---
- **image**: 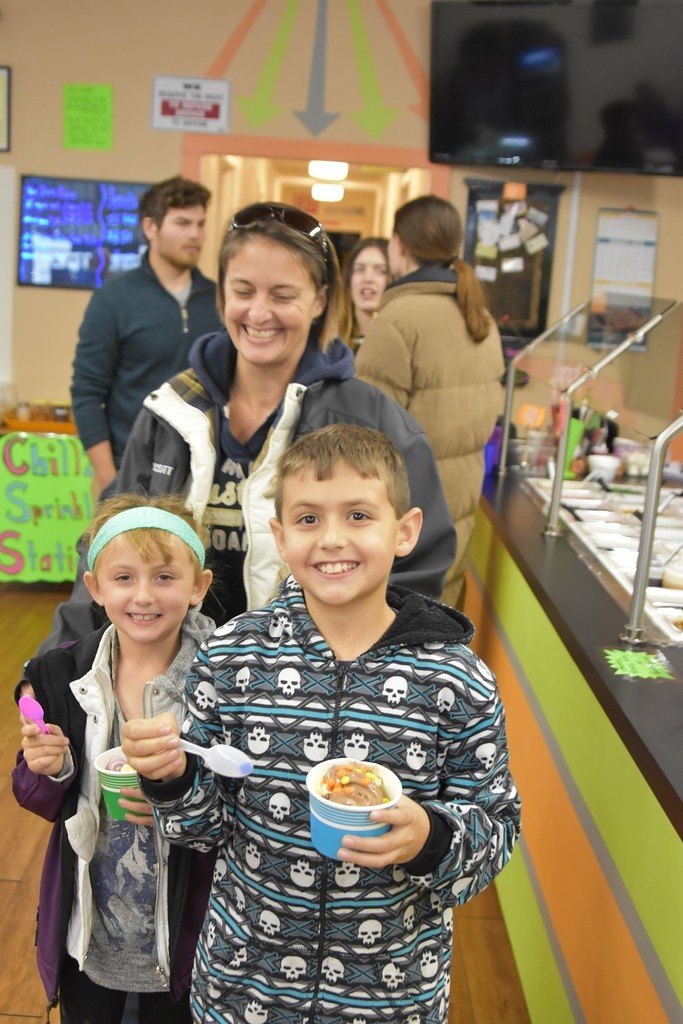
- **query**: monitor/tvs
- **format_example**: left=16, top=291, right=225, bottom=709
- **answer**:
left=428, top=0, right=683, bottom=177
left=17, top=175, right=155, bottom=291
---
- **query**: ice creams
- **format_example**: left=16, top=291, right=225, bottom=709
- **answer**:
left=321, top=761, right=390, bottom=807
left=105, top=756, right=137, bottom=774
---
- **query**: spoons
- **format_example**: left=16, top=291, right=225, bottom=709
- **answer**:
left=177, top=736, right=255, bottom=778
left=17, top=696, right=52, bottom=737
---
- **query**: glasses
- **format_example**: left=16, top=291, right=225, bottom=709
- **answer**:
left=226, top=206, right=330, bottom=270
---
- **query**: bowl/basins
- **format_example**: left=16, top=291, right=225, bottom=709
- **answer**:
left=305, top=758, right=402, bottom=862
left=93, top=746, right=150, bottom=822
left=588, top=454, right=620, bottom=481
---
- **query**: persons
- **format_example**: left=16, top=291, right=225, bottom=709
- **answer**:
left=121, top=423, right=524, bottom=1024
left=342, top=194, right=505, bottom=614
left=70, top=175, right=228, bottom=513
left=12, top=491, right=230, bottom=1024
left=38, top=201, right=458, bottom=659
